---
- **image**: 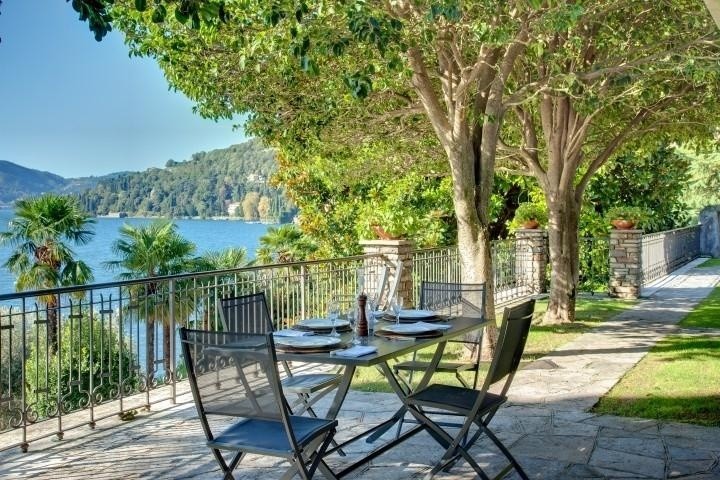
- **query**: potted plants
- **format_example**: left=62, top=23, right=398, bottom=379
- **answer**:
left=363, top=204, right=423, bottom=240
left=513, top=202, right=548, bottom=229
left=603, top=206, right=651, bottom=229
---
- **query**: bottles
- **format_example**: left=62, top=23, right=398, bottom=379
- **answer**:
left=355, top=275, right=373, bottom=338
left=357, top=295, right=368, bottom=345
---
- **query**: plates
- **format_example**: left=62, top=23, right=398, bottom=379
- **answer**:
left=275, top=345, right=347, bottom=355
left=298, top=319, right=350, bottom=330
left=292, top=327, right=352, bottom=333
left=384, top=324, right=431, bottom=335
left=389, top=310, right=436, bottom=319
left=374, top=330, right=443, bottom=338
left=383, top=316, right=442, bottom=322
left=276, top=336, right=342, bottom=349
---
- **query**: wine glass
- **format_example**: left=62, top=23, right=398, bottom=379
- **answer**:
left=328, top=308, right=341, bottom=337
left=393, top=297, right=404, bottom=326
